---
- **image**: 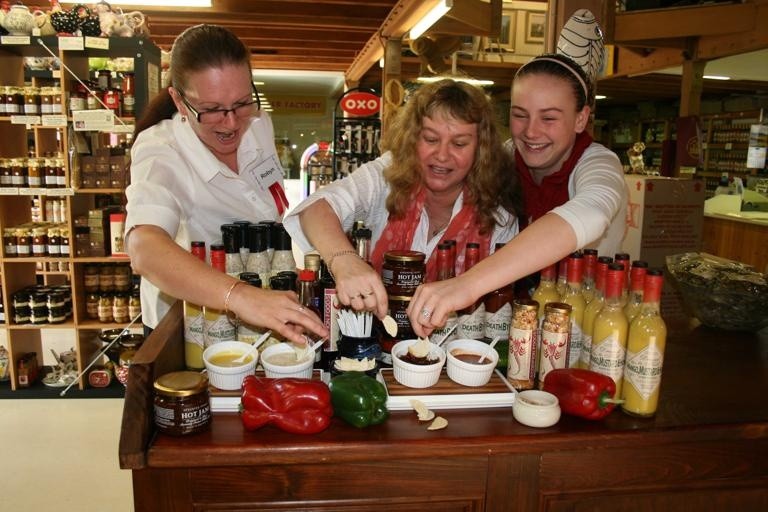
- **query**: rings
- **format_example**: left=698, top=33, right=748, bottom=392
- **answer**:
left=419, top=310, right=431, bottom=319
left=363, top=291, right=374, bottom=300
left=349, top=294, right=361, bottom=299
left=297, top=305, right=305, bottom=311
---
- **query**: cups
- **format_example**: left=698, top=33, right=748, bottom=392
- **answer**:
left=512, top=390, right=561, bottom=427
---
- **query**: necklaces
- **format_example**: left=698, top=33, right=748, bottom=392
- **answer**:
left=431, top=222, right=446, bottom=236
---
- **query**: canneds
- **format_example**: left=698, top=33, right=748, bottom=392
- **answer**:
left=154, top=370, right=212, bottom=436
left=380, top=249, right=427, bottom=354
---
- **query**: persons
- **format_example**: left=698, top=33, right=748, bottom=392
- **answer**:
left=405, top=53, right=630, bottom=341
left=122, top=22, right=329, bottom=348
left=280, top=77, right=520, bottom=321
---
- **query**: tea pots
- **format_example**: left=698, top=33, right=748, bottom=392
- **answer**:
left=0, top=2, right=145, bottom=36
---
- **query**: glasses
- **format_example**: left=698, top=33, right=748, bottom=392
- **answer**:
left=177, top=82, right=259, bottom=124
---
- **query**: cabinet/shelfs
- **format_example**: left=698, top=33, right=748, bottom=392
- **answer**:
left=607, top=108, right=767, bottom=199
left=0, top=35, right=161, bottom=398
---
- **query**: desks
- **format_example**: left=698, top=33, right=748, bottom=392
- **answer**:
left=118, top=298, right=764, bottom=511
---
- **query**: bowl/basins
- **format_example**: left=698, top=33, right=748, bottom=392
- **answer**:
left=202, top=341, right=317, bottom=390
left=390, top=340, right=499, bottom=388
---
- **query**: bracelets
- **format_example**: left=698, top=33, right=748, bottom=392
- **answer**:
left=225, top=281, right=245, bottom=314
left=327, top=250, right=362, bottom=284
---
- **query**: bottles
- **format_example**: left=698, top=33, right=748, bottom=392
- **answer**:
left=712, top=123, right=756, bottom=143
left=0, top=152, right=64, bottom=188
left=708, top=152, right=751, bottom=173
left=14, top=284, right=72, bottom=324
left=435, top=239, right=668, bottom=418
left=17, top=352, right=38, bottom=389
left=705, top=181, right=720, bottom=200
left=3, top=221, right=70, bottom=257
left=69, top=70, right=135, bottom=117
left=182, top=220, right=427, bottom=372
left=0, top=86, right=61, bottom=115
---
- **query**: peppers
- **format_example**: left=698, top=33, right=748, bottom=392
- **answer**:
left=327, top=371, right=387, bottom=427
left=238, top=375, right=333, bottom=435
left=543, top=368, right=627, bottom=421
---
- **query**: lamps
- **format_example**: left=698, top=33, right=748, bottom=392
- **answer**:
left=409, top=1, right=454, bottom=41
